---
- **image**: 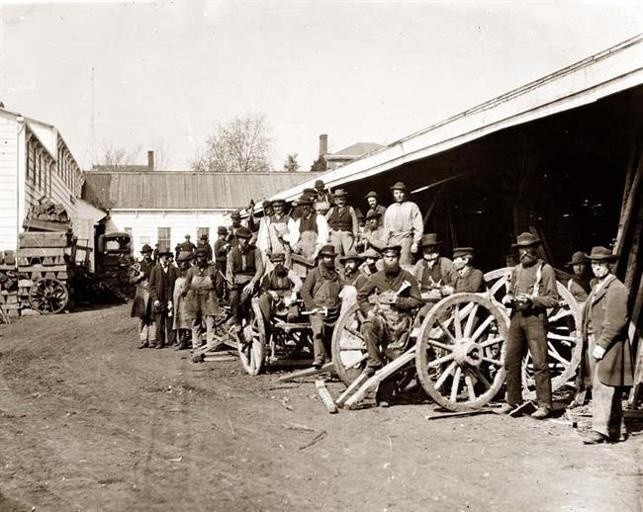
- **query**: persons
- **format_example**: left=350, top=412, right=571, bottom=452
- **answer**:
left=566, top=251, right=598, bottom=409
left=129, top=234, right=223, bottom=363
left=501, top=231, right=559, bottom=419
left=579, top=245, right=634, bottom=444
left=216, top=180, right=485, bottom=407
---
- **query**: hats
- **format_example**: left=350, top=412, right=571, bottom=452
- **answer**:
left=155, top=247, right=173, bottom=255
left=567, top=246, right=618, bottom=264
left=195, top=248, right=209, bottom=257
left=218, top=211, right=252, bottom=238
left=270, top=252, right=285, bottom=262
left=176, top=251, right=193, bottom=261
left=452, top=247, right=473, bottom=257
left=364, top=191, right=377, bottom=198
left=511, top=232, right=542, bottom=247
left=331, top=189, right=348, bottom=196
left=391, top=181, right=407, bottom=191
left=364, top=212, right=382, bottom=220
left=420, top=233, right=439, bottom=247
left=294, top=188, right=318, bottom=204
left=271, top=199, right=285, bottom=207
left=140, top=244, right=153, bottom=253
left=200, top=235, right=208, bottom=240
left=318, top=245, right=402, bottom=264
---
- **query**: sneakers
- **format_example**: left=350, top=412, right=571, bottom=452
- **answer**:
left=583, top=431, right=607, bottom=443
left=138, top=341, right=162, bottom=349
left=532, top=406, right=549, bottom=418
left=364, top=365, right=375, bottom=375
left=235, top=321, right=241, bottom=332
left=312, top=359, right=322, bottom=366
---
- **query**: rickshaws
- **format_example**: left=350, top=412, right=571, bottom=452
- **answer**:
left=189, top=266, right=584, bottom=415
